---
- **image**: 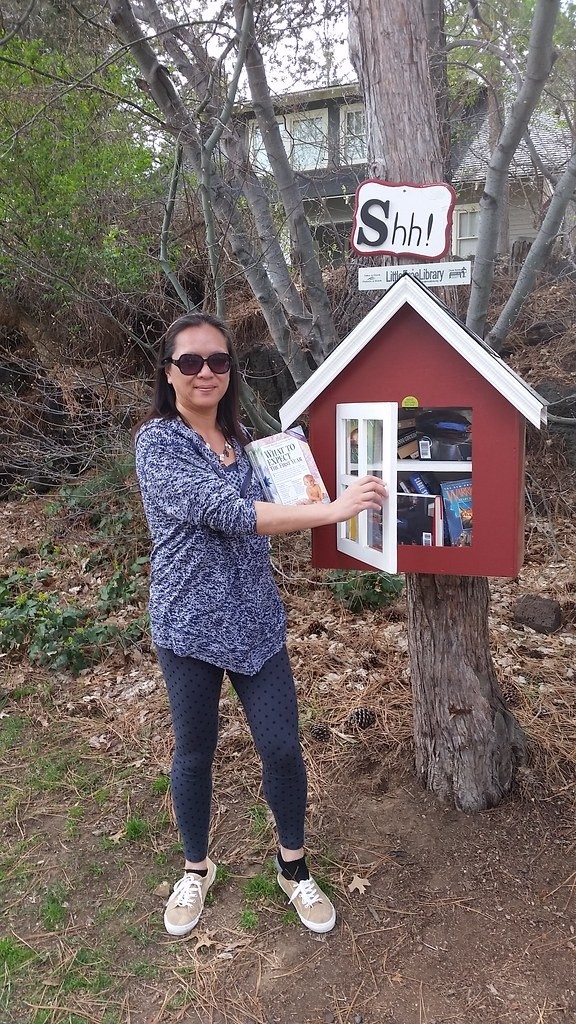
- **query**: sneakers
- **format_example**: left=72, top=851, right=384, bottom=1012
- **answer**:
left=163, top=856, right=216, bottom=936
left=276, top=872, right=336, bottom=933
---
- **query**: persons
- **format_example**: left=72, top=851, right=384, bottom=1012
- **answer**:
left=137, top=313, right=388, bottom=935
left=303, top=474, right=327, bottom=504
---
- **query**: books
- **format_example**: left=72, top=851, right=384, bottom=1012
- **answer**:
left=361, top=418, right=472, bottom=546
left=244, top=425, right=331, bottom=509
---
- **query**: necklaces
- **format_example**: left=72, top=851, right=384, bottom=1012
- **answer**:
left=195, top=431, right=231, bottom=462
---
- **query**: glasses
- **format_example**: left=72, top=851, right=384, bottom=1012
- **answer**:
left=167, top=354, right=232, bottom=376
